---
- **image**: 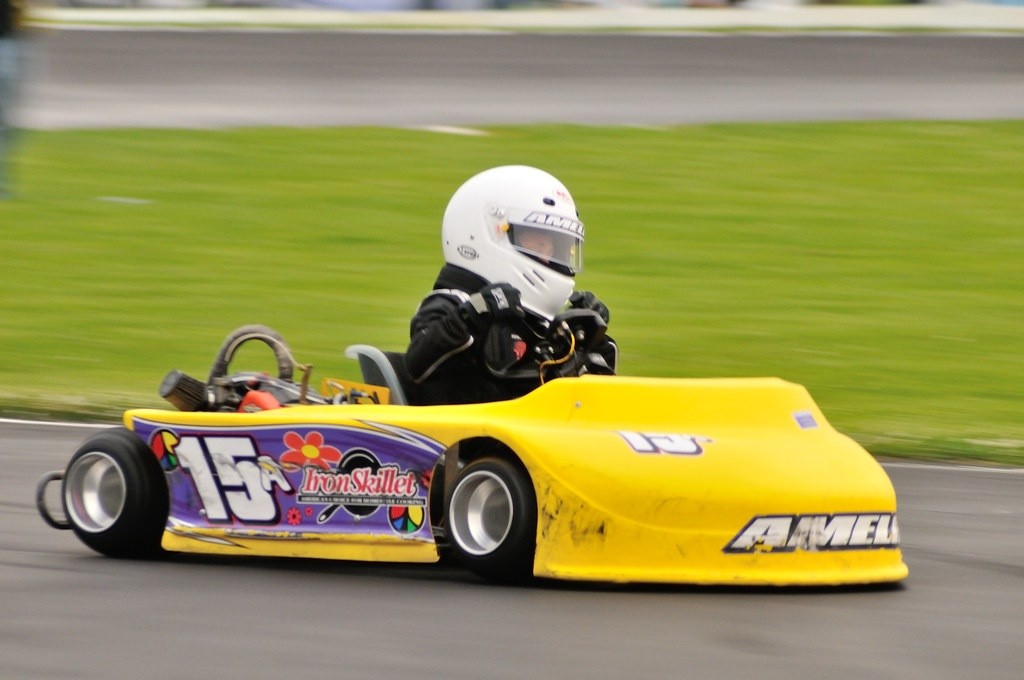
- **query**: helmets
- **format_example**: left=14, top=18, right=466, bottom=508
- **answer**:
left=441, top=163, right=586, bottom=323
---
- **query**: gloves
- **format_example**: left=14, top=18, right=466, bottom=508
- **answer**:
left=457, top=281, right=525, bottom=329
left=570, top=289, right=610, bottom=325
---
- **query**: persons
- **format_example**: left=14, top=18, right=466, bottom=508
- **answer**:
left=404, top=165, right=617, bottom=406
